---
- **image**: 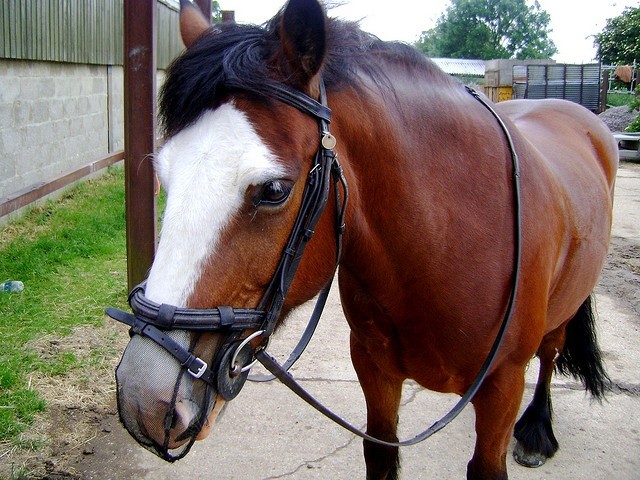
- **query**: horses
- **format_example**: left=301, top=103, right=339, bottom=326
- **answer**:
left=115, top=0, right=620, bottom=480
